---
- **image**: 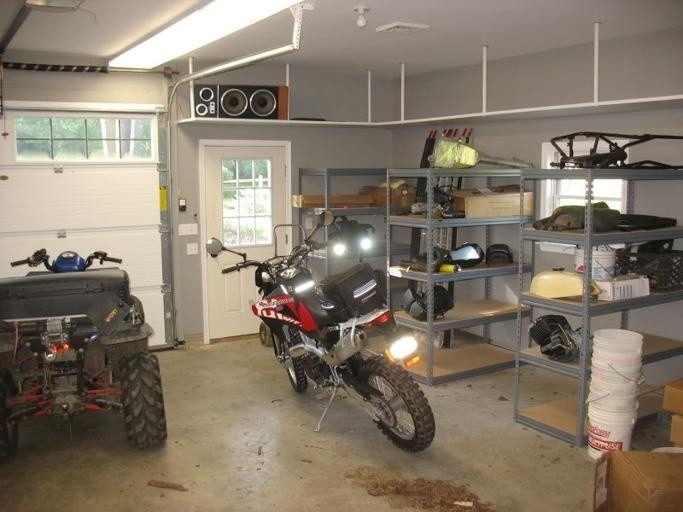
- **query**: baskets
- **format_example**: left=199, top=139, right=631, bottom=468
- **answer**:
left=615, top=247, right=683, bottom=293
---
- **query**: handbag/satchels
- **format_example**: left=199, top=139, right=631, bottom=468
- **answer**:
left=316, top=263, right=386, bottom=323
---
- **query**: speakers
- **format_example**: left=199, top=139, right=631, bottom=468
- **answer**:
left=194, top=84, right=288, bottom=119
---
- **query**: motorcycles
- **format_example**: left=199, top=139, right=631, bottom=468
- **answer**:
left=203, top=208, right=437, bottom=454
left=1, top=248, right=168, bottom=466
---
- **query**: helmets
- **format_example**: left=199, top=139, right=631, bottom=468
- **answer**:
left=421, top=283, right=454, bottom=316
left=485, top=244, right=513, bottom=267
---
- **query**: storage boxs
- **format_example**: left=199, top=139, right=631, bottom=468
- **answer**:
left=291, top=193, right=375, bottom=208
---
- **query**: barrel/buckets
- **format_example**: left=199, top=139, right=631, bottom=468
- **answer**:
left=585, top=327, right=646, bottom=461
left=574, top=248, right=616, bottom=280
